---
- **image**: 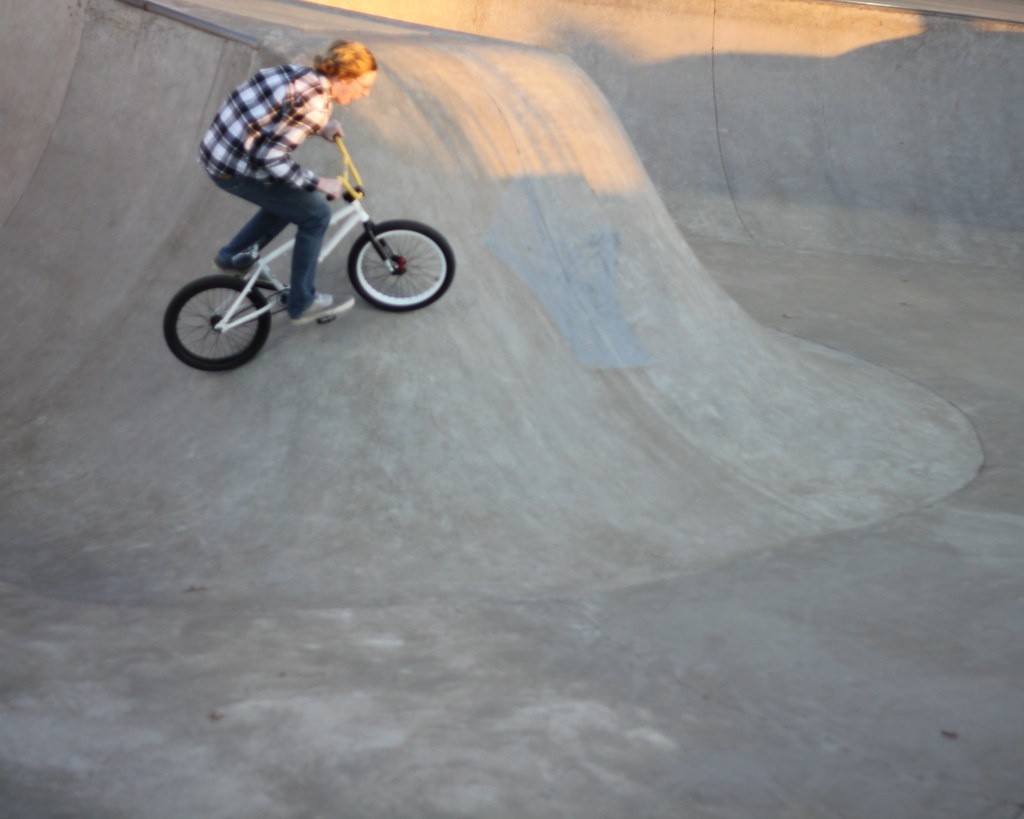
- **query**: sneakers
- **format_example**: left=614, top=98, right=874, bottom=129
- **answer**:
left=212, top=257, right=277, bottom=281
left=289, top=291, right=355, bottom=325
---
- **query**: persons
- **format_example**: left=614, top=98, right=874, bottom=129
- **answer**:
left=198, top=39, right=378, bottom=325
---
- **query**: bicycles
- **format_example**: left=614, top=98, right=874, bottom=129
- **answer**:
left=163, top=133, right=456, bottom=371
